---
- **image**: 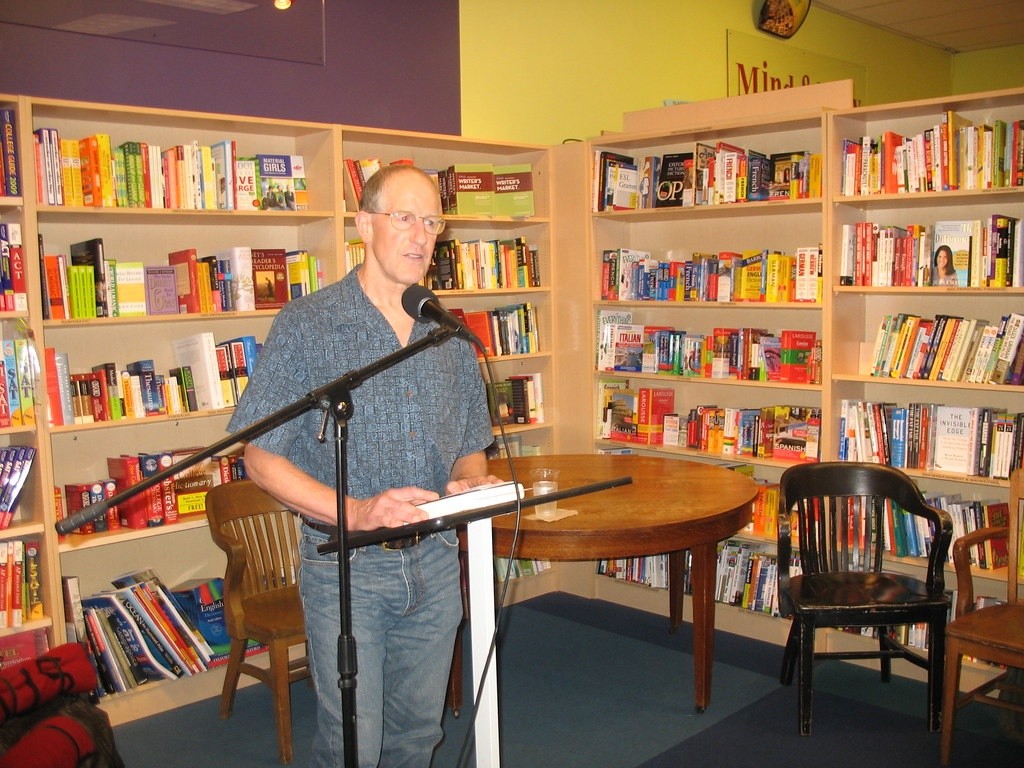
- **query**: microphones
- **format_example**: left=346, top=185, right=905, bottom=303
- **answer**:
left=401, top=285, right=485, bottom=351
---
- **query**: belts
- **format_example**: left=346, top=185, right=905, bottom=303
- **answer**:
left=302, top=517, right=432, bottom=550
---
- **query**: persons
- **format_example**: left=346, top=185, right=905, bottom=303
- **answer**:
left=226, top=164, right=505, bottom=768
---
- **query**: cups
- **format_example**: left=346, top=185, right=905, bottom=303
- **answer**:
left=530, top=468, right=560, bottom=518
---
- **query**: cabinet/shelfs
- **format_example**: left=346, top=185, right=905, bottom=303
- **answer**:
left=825, top=85, right=1024, bottom=677
left=1, top=90, right=59, bottom=677
left=586, top=78, right=859, bottom=663
left=338, top=124, right=587, bottom=607
left=28, top=97, right=347, bottom=728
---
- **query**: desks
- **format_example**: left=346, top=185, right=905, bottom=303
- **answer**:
left=440, top=452, right=762, bottom=723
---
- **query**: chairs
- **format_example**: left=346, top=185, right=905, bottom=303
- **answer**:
left=943, top=468, right=1024, bottom=768
left=775, top=460, right=955, bottom=738
left=204, top=477, right=317, bottom=765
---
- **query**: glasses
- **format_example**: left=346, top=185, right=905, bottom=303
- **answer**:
left=369, top=211, right=448, bottom=236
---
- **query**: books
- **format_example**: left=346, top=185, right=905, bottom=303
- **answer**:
left=0, top=567, right=269, bottom=672
left=0, top=109, right=323, bottom=626
left=343, top=158, right=545, bottom=460
left=591, top=141, right=823, bottom=621
left=837, top=110, right=1024, bottom=668
